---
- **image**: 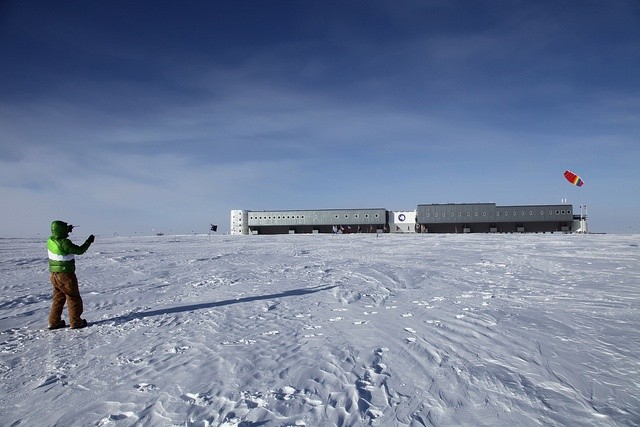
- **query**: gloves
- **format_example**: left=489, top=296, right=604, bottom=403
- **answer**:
left=87, top=235, right=94, bottom=243
left=69, top=225, right=72, bottom=232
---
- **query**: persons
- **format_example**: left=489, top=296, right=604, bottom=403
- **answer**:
left=47, top=220, right=96, bottom=329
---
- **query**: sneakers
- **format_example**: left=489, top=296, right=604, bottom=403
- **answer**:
left=69, top=319, right=86, bottom=329
left=47, top=320, right=65, bottom=329
